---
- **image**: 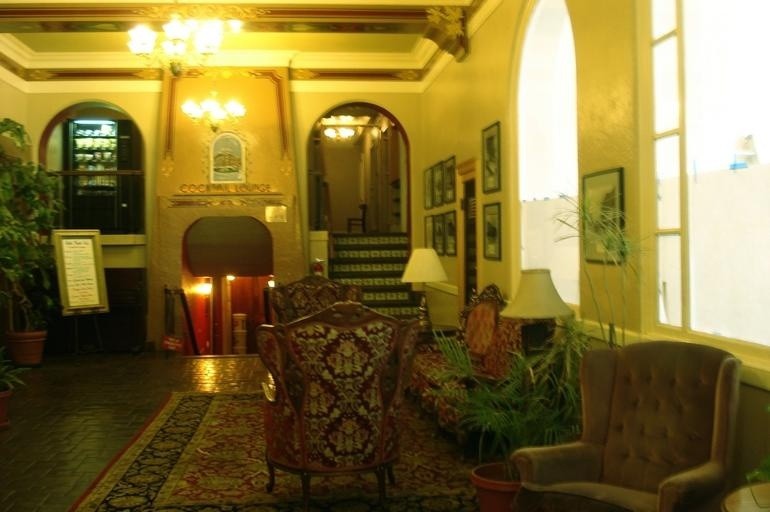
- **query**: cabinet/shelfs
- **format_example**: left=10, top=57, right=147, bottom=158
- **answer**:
left=64, top=117, right=133, bottom=234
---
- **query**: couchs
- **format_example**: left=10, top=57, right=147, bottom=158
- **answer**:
left=408, top=283, right=537, bottom=447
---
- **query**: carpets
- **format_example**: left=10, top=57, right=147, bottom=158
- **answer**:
left=65, top=391, right=507, bottom=512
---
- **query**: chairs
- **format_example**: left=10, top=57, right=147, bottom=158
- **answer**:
left=347, top=204, right=367, bottom=234
left=263, top=274, right=364, bottom=326
left=509, top=339, right=743, bottom=512
left=253, top=300, right=421, bottom=512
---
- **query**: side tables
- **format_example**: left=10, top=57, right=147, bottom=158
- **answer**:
left=415, top=319, right=459, bottom=348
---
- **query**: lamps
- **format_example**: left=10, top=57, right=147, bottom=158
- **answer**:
left=126, top=20, right=217, bottom=78
left=176, top=90, right=245, bottom=133
left=400, top=248, right=448, bottom=328
left=498, top=269, right=576, bottom=357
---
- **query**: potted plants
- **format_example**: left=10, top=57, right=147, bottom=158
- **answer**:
left=0, top=346, right=32, bottom=430
left=430, top=317, right=590, bottom=511
left=0, top=117, right=67, bottom=366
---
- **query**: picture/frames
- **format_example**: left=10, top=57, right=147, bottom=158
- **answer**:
left=582, top=166, right=625, bottom=266
left=482, top=201, right=502, bottom=261
left=209, top=132, right=247, bottom=184
left=480, top=120, right=501, bottom=194
left=423, top=155, right=457, bottom=255
